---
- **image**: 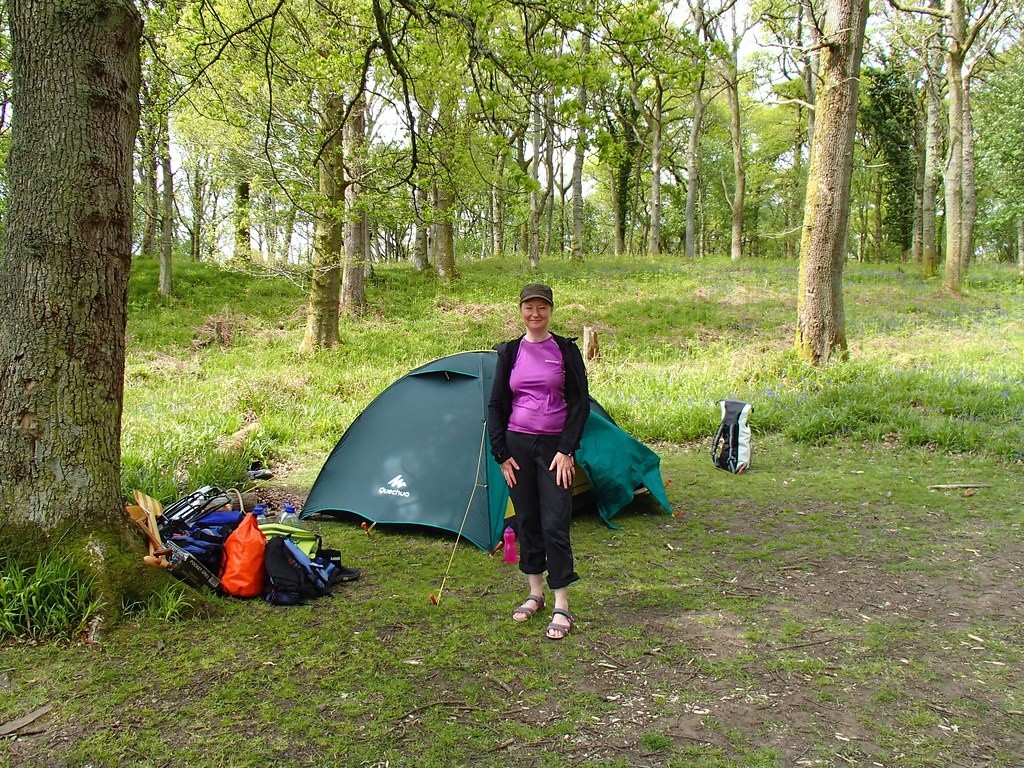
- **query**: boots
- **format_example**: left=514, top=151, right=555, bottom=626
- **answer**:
left=314, top=548, right=360, bottom=583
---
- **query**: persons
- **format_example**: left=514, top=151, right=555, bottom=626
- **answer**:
left=488, top=283, right=591, bottom=639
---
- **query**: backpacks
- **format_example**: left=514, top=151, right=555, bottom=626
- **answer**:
left=265, top=533, right=329, bottom=605
left=713, top=399, right=754, bottom=475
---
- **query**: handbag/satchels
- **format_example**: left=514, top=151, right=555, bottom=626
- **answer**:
left=217, top=512, right=267, bottom=598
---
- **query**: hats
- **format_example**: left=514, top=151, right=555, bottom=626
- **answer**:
left=520, top=283, right=553, bottom=305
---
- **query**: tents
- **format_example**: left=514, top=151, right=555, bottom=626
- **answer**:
left=298, top=351, right=676, bottom=555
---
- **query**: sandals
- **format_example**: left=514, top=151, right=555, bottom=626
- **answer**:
left=546, top=608, right=573, bottom=639
left=512, top=591, right=546, bottom=622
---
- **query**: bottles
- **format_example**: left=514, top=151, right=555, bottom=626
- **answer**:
left=253, top=508, right=266, bottom=525
left=256, top=504, right=269, bottom=524
left=504, top=526, right=517, bottom=563
left=279, top=506, right=302, bottom=529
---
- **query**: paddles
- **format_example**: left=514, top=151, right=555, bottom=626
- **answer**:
left=125, top=490, right=174, bottom=571
